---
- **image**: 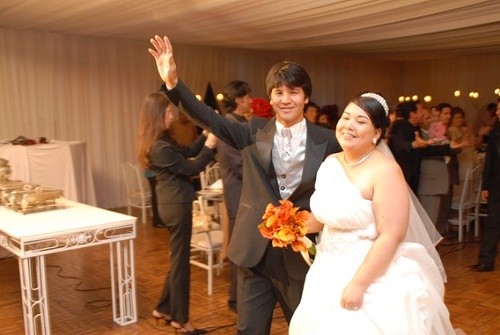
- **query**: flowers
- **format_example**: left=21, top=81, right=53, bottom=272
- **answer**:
left=258, top=199, right=316, bottom=267
left=251, top=97, right=273, bottom=120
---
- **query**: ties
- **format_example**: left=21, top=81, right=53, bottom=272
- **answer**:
left=279, top=127, right=292, bottom=166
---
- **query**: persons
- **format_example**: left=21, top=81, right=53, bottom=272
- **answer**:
left=148, top=35, right=343, bottom=335
left=136, top=91, right=220, bottom=335
left=144, top=168, right=168, bottom=228
left=384, top=97, right=500, bottom=271
left=304, top=102, right=340, bottom=131
left=290, top=96, right=435, bottom=335
left=213, top=79, right=253, bottom=312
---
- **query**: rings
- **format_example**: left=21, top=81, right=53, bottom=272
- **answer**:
left=353, top=305, right=359, bottom=310
left=345, top=306, right=352, bottom=310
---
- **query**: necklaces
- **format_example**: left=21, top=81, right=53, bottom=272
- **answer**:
left=341, top=148, right=374, bottom=168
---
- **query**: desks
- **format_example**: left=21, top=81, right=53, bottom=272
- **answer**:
left=0, top=198, right=138, bottom=335
left=0, top=140, right=97, bottom=208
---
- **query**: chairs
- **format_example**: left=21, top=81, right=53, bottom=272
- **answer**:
left=448, top=165, right=482, bottom=242
left=120, top=161, right=154, bottom=224
left=189, top=161, right=231, bottom=296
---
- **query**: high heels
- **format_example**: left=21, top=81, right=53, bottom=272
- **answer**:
left=151, top=310, right=173, bottom=326
left=173, top=322, right=207, bottom=335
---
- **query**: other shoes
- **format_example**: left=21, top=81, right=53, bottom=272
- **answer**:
left=468, top=261, right=494, bottom=272
